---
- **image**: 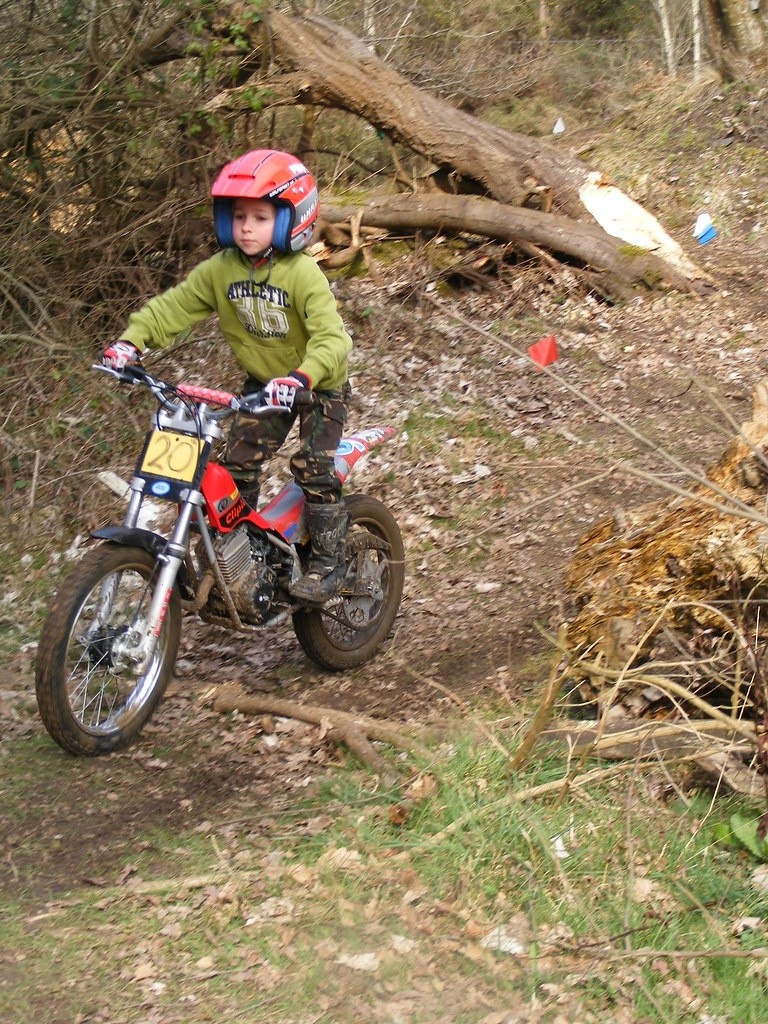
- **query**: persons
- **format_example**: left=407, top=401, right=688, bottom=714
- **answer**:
left=103, top=150, right=353, bottom=602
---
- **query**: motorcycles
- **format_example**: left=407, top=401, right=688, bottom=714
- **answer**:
left=30, top=351, right=408, bottom=755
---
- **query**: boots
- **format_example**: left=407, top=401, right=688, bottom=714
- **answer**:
left=290, top=502, right=351, bottom=603
left=241, top=488, right=263, bottom=506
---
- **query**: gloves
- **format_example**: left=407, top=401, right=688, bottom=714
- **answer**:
left=103, top=339, right=140, bottom=374
left=263, top=369, right=311, bottom=413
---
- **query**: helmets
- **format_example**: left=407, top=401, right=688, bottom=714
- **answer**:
left=211, top=150, right=317, bottom=251
